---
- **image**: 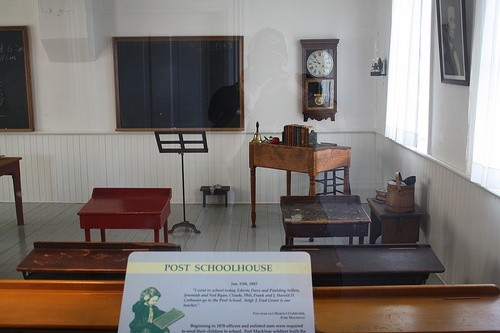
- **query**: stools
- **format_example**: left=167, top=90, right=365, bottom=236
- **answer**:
left=200, top=186, right=230, bottom=207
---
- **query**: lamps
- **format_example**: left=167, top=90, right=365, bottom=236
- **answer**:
left=370, top=57, right=386, bottom=76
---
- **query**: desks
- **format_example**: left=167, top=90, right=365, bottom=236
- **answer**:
left=280, top=244, right=445, bottom=287
left=16, top=242, right=181, bottom=280
left=248, top=142, right=352, bottom=242
left=366, top=198, right=425, bottom=244
left=0, top=157, right=25, bottom=225
left=0, top=279, right=500, bottom=333
left=77, top=188, right=173, bottom=243
left=279, top=195, right=372, bottom=246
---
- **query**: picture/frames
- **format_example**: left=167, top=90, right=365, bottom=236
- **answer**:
left=436, top=0, right=468, bottom=86
left=0, top=25, right=35, bottom=132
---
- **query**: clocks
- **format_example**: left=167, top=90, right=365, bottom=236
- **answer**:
left=300, top=39, right=340, bottom=122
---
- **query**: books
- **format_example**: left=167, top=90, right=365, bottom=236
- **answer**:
left=282, top=123, right=317, bottom=147
